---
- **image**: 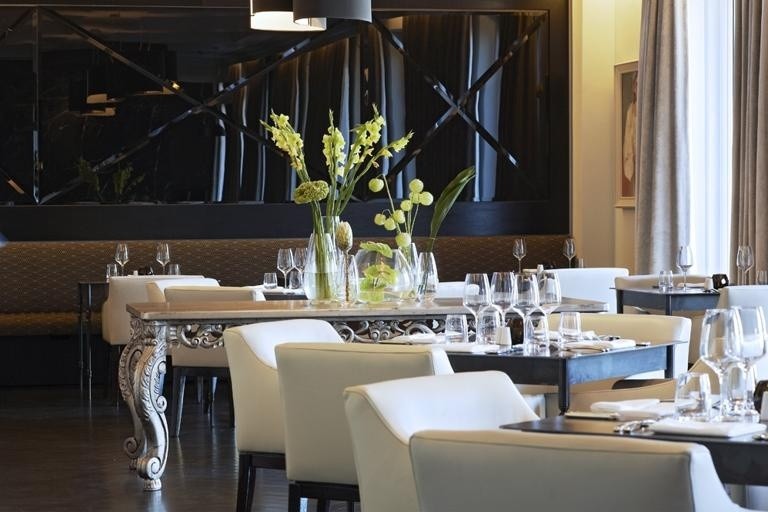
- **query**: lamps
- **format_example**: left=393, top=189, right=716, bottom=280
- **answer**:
left=248, top=0, right=375, bottom=41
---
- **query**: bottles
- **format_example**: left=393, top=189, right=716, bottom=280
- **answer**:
left=759, top=389, right=768, bottom=423
left=545, top=263, right=561, bottom=269
left=578, top=258, right=584, bottom=268
left=133, top=266, right=154, bottom=276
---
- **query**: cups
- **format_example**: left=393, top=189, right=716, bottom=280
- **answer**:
left=262, top=272, right=277, bottom=289
left=535, top=263, right=544, bottom=271
left=658, top=270, right=672, bottom=289
left=754, top=271, right=767, bottom=285
left=494, top=326, right=513, bottom=345
left=475, top=305, right=500, bottom=345
left=669, top=370, right=712, bottom=422
left=106, top=262, right=117, bottom=279
left=557, top=310, right=581, bottom=346
left=702, top=278, right=713, bottom=291
left=524, top=313, right=551, bottom=353
left=167, top=263, right=180, bottom=276
left=289, top=270, right=301, bottom=291
left=444, top=314, right=468, bottom=345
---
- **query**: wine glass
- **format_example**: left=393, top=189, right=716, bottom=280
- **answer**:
left=462, top=273, right=492, bottom=347
left=115, top=242, right=129, bottom=277
left=533, top=270, right=560, bottom=353
left=292, top=247, right=309, bottom=292
left=736, top=245, right=753, bottom=285
left=561, top=237, right=577, bottom=268
left=673, top=245, right=693, bottom=291
left=509, top=274, right=536, bottom=353
left=489, top=270, right=515, bottom=352
left=155, top=242, right=170, bottom=274
left=697, top=306, right=764, bottom=425
left=276, top=247, right=292, bottom=292
left=511, top=238, right=527, bottom=273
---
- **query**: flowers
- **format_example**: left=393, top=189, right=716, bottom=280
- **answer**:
left=265, top=107, right=478, bottom=231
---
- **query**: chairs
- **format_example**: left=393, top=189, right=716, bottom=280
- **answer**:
left=1, top=234, right=767, bottom=511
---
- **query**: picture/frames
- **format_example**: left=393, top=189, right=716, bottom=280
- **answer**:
left=611, top=58, right=637, bottom=212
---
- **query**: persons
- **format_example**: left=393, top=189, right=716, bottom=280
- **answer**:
left=621, top=71, right=638, bottom=199
